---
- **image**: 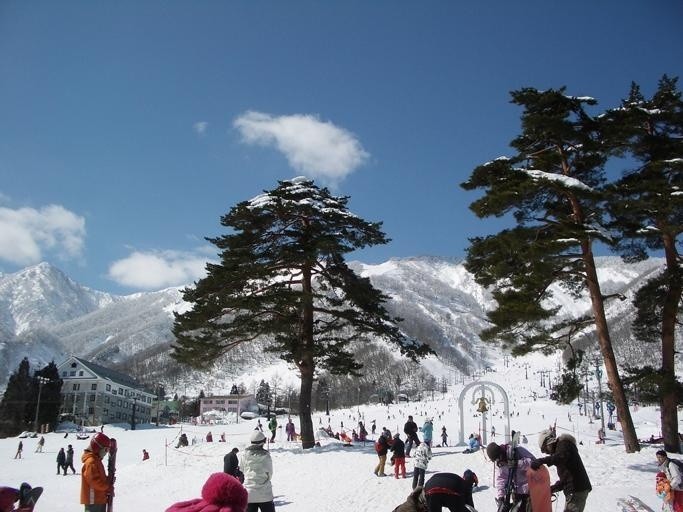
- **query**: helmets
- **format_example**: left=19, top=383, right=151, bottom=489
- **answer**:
left=90, top=432, right=111, bottom=457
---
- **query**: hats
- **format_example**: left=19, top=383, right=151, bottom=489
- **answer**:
left=538, top=429, right=555, bottom=452
left=487, top=443, right=501, bottom=462
left=250, top=430, right=266, bottom=446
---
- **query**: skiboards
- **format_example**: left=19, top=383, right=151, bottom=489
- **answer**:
left=617, top=496, right=657, bottom=511
left=107, top=438, right=117, bottom=511
left=18, top=483, right=43, bottom=511
left=497, top=431, right=522, bottom=512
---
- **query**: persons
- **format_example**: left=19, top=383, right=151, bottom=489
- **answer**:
left=224, top=448, right=245, bottom=484
left=165, top=472, right=248, bottom=512
left=650, top=435, right=654, bottom=442
left=270, top=415, right=277, bottom=443
left=206, top=432, right=212, bottom=442
left=0, top=432, right=116, bottom=512
left=175, top=434, right=188, bottom=448
left=239, top=430, right=275, bottom=512
left=318, top=415, right=592, bottom=512
left=142, top=449, right=149, bottom=460
left=286, top=419, right=295, bottom=441
left=656, top=450, right=683, bottom=512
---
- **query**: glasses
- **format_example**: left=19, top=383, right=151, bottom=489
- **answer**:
left=92, top=436, right=110, bottom=453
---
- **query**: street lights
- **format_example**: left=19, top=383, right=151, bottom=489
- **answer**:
left=156, top=384, right=162, bottom=426
left=33, top=376, right=52, bottom=431
left=131, top=398, right=137, bottom=431
left=357, top=388, right=360, bottom=412
left=325, top=393, right=329, bottom=415
